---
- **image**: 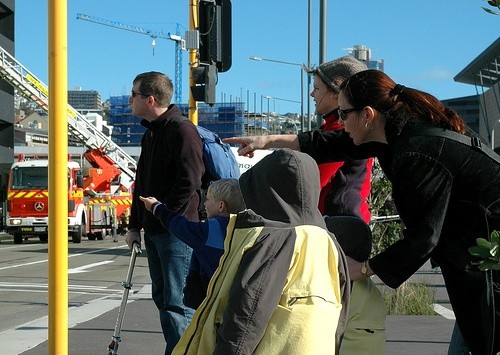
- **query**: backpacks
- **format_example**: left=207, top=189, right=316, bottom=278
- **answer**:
left=167, top=115, right=240, bottom=188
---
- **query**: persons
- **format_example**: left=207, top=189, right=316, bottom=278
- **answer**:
left=302, top=57, right=374, bottom=224
left=323, top=215, right=388, bottom=355
left=125, top=73, right=206, bottom=354
left=222, top=70, right=500, bottom=355
left=139, top=179, right=246, bottom=310
left=171, top=148, right=351, bottom=355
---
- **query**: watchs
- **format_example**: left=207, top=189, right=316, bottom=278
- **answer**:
left=361, top=261, right=368, bottom=278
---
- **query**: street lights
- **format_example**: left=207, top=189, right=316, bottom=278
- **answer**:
left=261, top=94, right=271, bottom=134
left=249, top=56, right=304, bottom=133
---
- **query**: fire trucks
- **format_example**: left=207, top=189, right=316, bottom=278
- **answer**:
left=0, top=45, right=139, bottom=245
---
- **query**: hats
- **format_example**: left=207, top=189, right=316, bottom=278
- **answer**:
left=317, top=54, right=369, bottom=91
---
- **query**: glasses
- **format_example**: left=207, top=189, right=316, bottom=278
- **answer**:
left=132, top=90, right=159, bottom=103
left=337, top=108, right=361, bottom=120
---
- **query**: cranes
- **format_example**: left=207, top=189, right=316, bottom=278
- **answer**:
left=76, top=11, right=189, bottom=110
